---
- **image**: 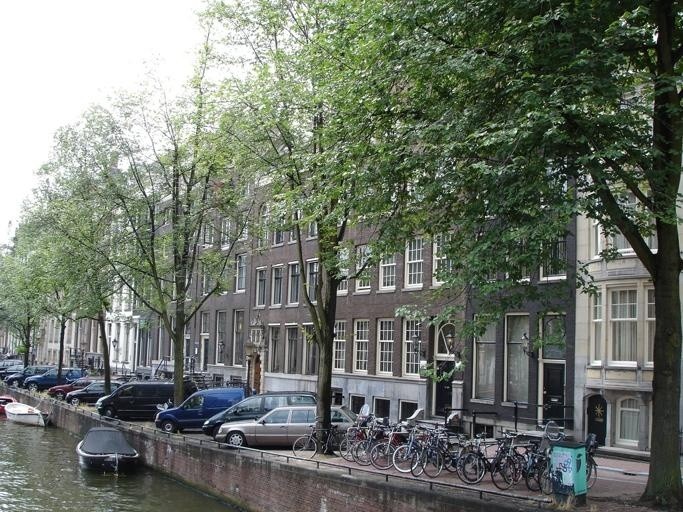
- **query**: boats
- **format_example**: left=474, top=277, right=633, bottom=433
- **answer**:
left=74, top=426, right=139, bottom=475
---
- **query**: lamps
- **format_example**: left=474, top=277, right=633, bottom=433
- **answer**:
left=445, top=331, right=463, bottom=360
left=112, top=338, right=118, bottom=353
left=412, top=332, right=426, bottom=358
left=521, top=333, right=535, bottom=359
left=218, top=340, right=225, bottom=354
